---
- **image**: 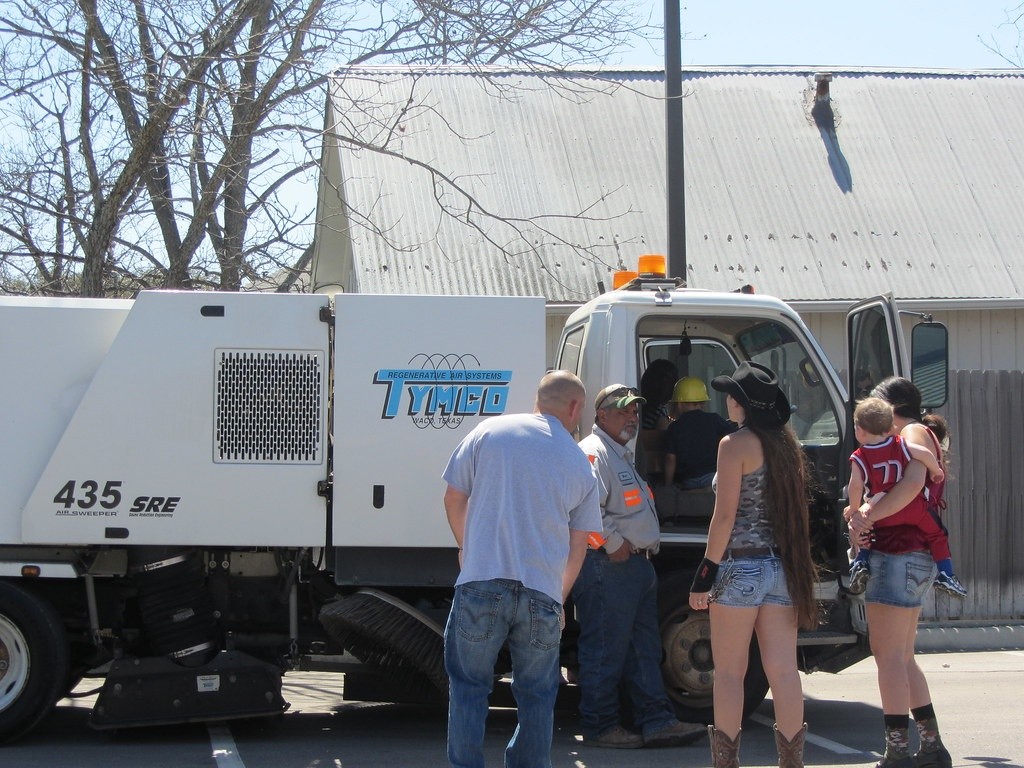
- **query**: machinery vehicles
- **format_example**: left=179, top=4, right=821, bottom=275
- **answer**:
left=0, top=255, right=948, bottom=735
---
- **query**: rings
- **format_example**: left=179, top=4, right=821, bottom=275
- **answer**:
left=697, top=600, right=701, bottom=604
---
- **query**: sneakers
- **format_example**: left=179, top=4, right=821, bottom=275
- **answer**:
left=932, top=570, right=968, bottom=600
left=849, top=560, right=870, bottom=594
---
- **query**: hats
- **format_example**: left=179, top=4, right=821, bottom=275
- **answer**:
left=594, top=383, right=647, bottom=415
left=711, top=360, right=792, bottom=429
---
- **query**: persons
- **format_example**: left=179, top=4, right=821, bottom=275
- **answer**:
left=573, top=384, right=706, bottom=748
left=688, top=361, right=823, bottom=768
left=443, top=369, right=604, bottom=768
left=843, top=378, right=967, bottom=768
left=639, top=359, right=738, bottom=495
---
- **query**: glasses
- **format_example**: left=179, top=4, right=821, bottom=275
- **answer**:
left=596, top=387, right=641, bottom=410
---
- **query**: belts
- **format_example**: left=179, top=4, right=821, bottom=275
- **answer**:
left=587, top=543, right=653, bottom=560
left=721, top=547, right=781, bottom=561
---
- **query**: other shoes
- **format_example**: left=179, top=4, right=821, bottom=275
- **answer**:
left=874, top=755, right=912, bottom=768
left=583, top=725, right=644, bottom=749
left=643, top=721, right=707, bottom=748
left=912, top=749, right=952, bottom=768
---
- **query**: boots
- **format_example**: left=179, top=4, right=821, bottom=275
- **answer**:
left=707, top=724, right=742, bottom=768
left=773, top=722, right=808, bottom=768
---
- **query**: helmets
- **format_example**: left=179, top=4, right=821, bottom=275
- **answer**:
left=668, top=375, right=712, bottom=403
